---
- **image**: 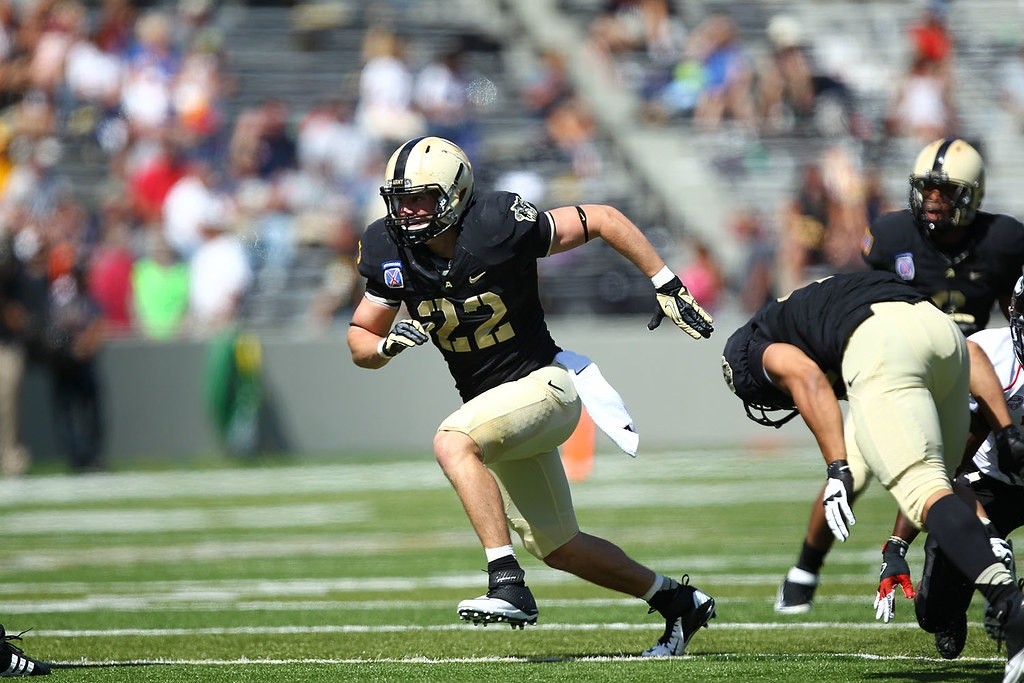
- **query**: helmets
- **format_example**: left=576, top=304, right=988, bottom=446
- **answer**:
left=1008, top=275, right=1024, bottom=363
left=908, top=138, right=986, bottom=228
left=380, top=135, right=475, bottom=248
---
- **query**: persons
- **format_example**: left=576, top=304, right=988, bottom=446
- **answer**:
left=873, top=275, right=1024, bottom=683
left=329, top=26, right=721, bottom=314
left=346, top=134, right=715, bottom=655
left=774, top=133, right=1024, bottom=614
left=602, top=0, right=955, bottom=307
left=722, top=272, right=1024, bottom=683
left=0, top=0, right=352, bottom=479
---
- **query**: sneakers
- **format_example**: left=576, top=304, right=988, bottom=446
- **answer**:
left=985, top=560, right=1024, bottom=683
left=935, top=610, right=968, bottom=659
left=456, top=566, right=538, bottom=630
left=774, top=566, right=818, bottom=615
left=0, top=627, right=51, bottom=676
left=984, top=538, right=1017, bottom=643
left=642, top=574, right=717, bottom=657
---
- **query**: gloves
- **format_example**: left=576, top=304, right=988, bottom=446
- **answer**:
left=995, top=424, right=1024, bottom=485
left=824, top=459, right=856, bottom=542
left=382, top=319, right=435, bottom=357
left=874, top=536, right=916, bottom=623
left=648, top=274, right=714, bottom=340
